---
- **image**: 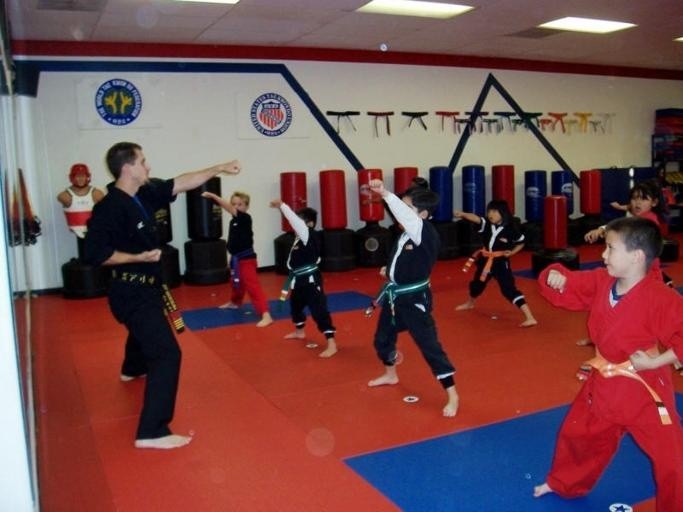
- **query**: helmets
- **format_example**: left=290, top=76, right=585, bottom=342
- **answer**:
left=69, top=164, right=90, bottom=188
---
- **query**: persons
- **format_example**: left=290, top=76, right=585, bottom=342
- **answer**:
left=269, top=199, right=337, bottom=358
left=54, top=164, right=104, bottom=240
left=608, top=175, right=682, bottom=217
left=80, top=141, right=239, bottom=449
left=361, top=177, right=427, bottom=236
left=532, top=216, right=682, bottom=511
left=576, top=178, right=682, bottom=373
left=364, top=178, right=458, bottom=417
left=451, top=199, right=537, bottom=328
left=200, top=190, right=273, bottom=328
